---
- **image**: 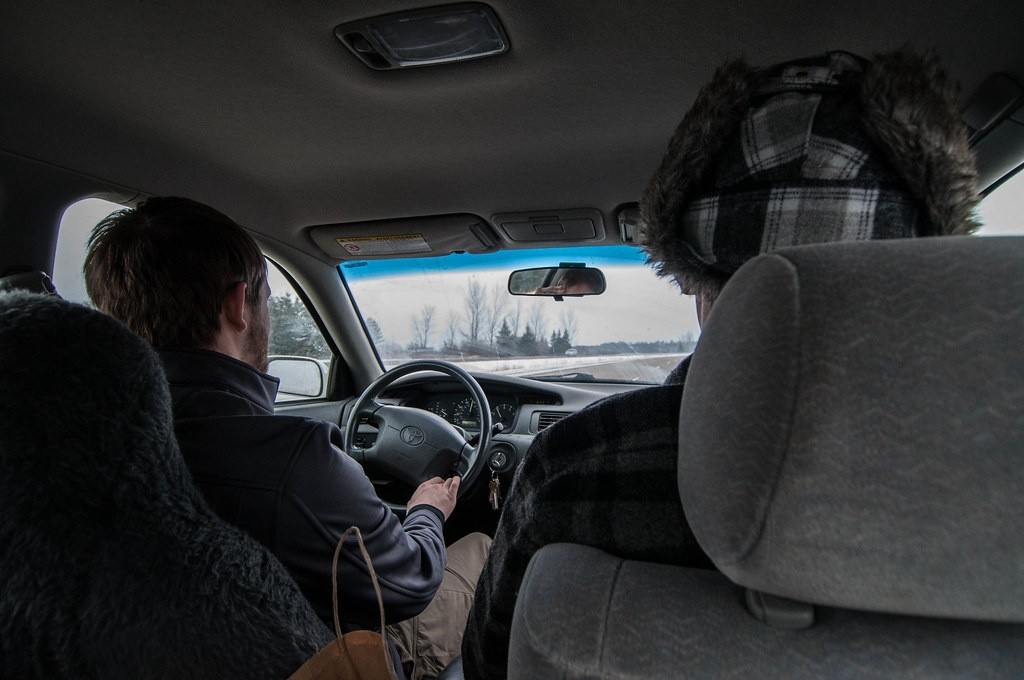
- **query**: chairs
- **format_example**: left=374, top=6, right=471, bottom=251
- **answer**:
left=505, top=236, right=1024, bottom=678
left=0, top=287, right=336, bottom=680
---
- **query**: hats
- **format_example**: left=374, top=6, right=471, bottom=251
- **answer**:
left=634, top=45, right=985, bottom=302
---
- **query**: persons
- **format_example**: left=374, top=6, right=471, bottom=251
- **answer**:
left=533, top=267, right=603, bottom=294
left=459, top=43, right=985, bottom=678
left=82, top=197, right=497, bottom=680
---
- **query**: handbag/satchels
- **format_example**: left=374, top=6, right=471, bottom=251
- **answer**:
left=289, top=527, right=402, bottom=679
